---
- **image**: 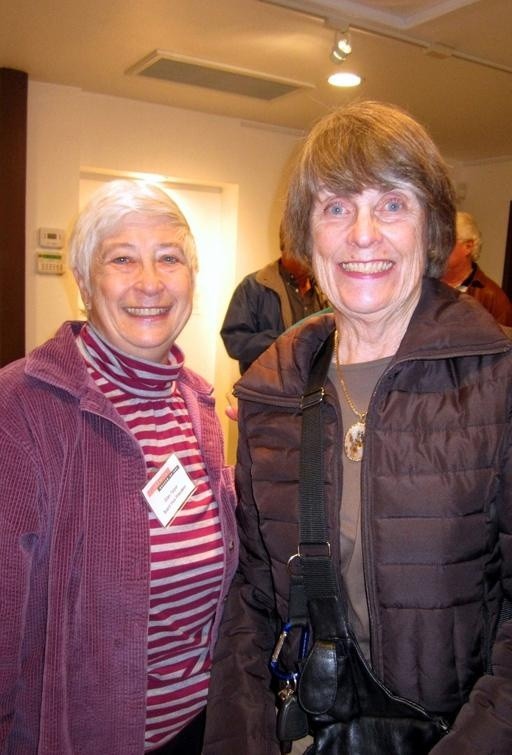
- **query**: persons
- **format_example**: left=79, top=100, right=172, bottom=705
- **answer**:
left=220, top=218, right=332, bottom=378
left=0, top=174, right=243, bottom=754
left=437, top=212, right=511, bottom=343
left=197, top=99, right=512, bottom=755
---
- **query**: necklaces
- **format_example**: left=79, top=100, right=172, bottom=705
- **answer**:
left=333, top=330, right=368, bottom=464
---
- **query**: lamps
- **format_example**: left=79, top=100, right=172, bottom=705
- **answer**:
left=328, top=29, right=352, bottom=66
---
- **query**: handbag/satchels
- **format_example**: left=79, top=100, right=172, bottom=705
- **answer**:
left=268, top=326, right=453, bottom=755
